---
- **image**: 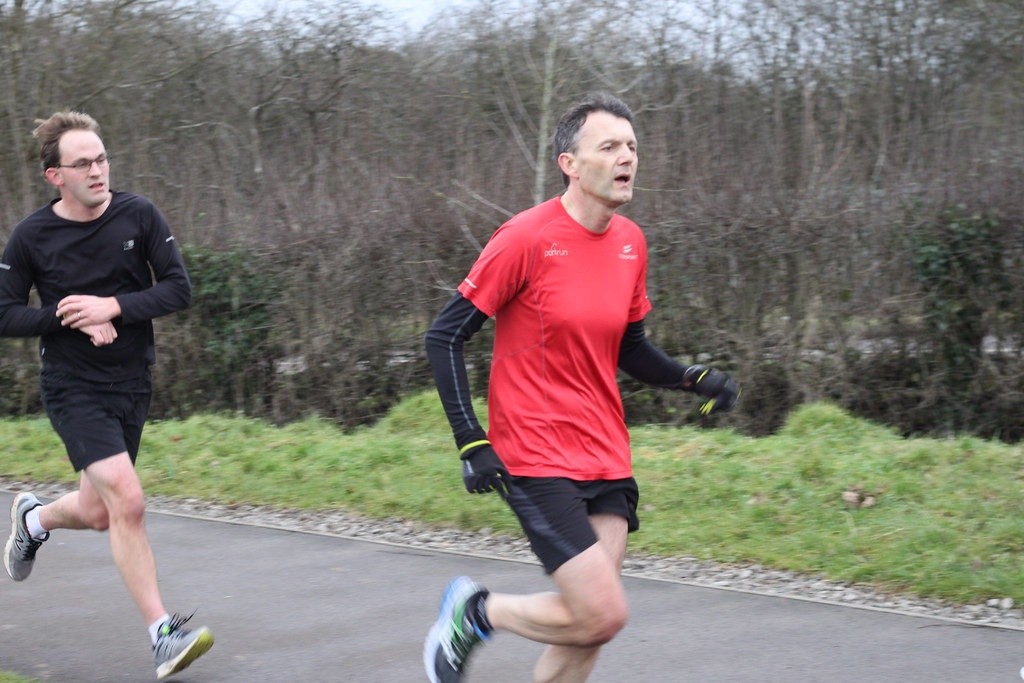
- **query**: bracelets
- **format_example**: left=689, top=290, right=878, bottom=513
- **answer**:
left=62, top=312, right=69, bottom=327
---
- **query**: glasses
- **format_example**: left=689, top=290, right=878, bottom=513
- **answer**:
left=59, top=153, right=111, bottom=172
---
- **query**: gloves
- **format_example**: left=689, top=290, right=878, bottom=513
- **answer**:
left=456, top=430, right=515, bottom=500
left=683, top=364, right=741, bottom=416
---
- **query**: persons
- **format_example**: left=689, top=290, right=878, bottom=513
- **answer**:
left=421, top=90, right=747, bottom=683
left=0, top=107, right=216, bottom=681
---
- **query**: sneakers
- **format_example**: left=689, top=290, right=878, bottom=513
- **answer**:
left=153, top=609, right=214, bottom=680
left=423, top=575, right=495, bottom=682
left=3, top=492, right=50, bottom=582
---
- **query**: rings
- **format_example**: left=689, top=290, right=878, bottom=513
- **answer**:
left=77, top=312, right=81, bottom=320
